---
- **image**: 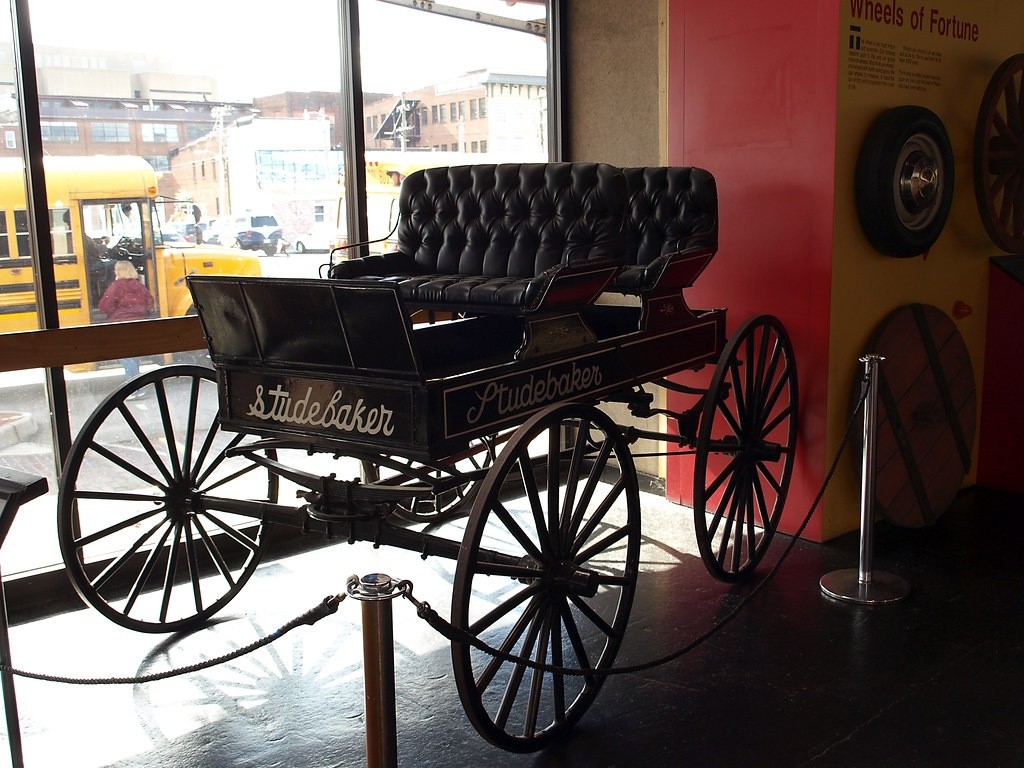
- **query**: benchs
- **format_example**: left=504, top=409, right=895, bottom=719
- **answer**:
left=320, top=161, right=720, bottom=363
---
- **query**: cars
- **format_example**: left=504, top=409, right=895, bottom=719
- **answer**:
left=292, top=226, right=335, bottom=254
left=163, top=213, right=283, bottom=255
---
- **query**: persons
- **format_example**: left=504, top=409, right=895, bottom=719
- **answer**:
left=62, top=210, right=117, bottom=287
left=98, top=260, right=155, bottom=400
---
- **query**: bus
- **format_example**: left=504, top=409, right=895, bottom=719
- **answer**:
left=0, top=154, right=263, bottom=374
